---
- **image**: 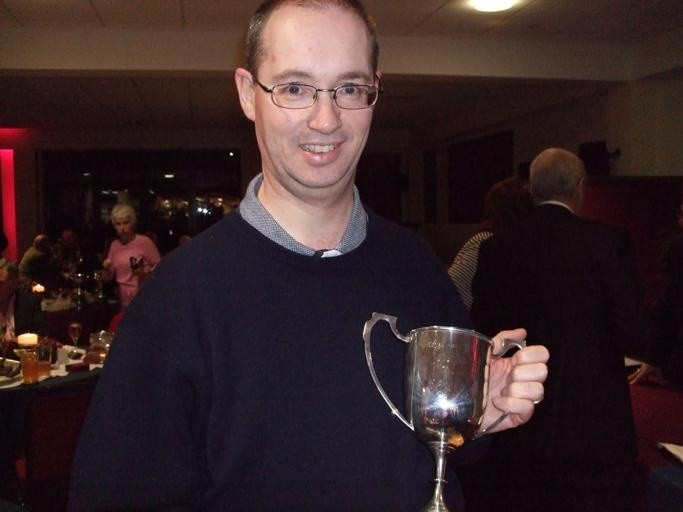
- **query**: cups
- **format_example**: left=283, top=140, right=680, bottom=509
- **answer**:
left=18, top=349, right=40, bottom=384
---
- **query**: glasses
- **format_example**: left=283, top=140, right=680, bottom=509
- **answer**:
left=252, top=76, right=380, bottom=111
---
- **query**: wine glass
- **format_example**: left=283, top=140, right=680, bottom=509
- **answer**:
left=68, top=325, right=81, bottom=350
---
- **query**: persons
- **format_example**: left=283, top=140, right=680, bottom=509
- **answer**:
left=0, top=202, right=193, bottom=343
left=471, top=145, right=647, bottom=512
left=447, top=176, right=533, bottom=312
left=68, top=0, right=551, bottom=512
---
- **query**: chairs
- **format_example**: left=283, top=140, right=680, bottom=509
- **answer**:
left=14, top=391, right=94, bottom=512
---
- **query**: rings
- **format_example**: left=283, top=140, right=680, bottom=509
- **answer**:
left=532, top=395, right=545, bottom=405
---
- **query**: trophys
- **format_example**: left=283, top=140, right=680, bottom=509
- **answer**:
left=361, top=310, right=527, bottom=512
